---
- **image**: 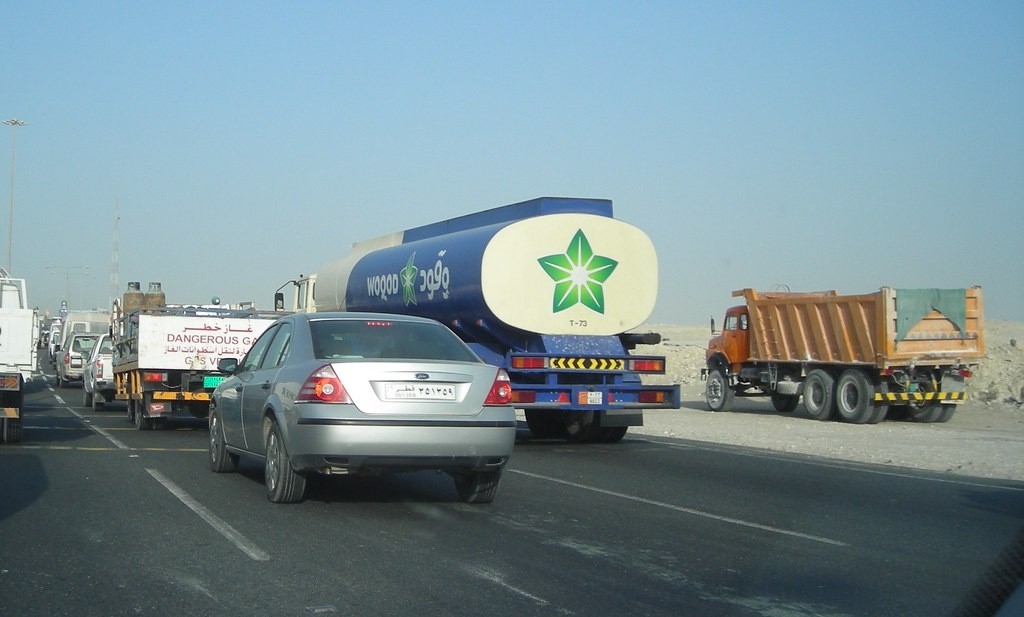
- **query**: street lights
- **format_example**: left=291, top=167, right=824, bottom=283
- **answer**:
left=6, top=114, right=29, bottom=276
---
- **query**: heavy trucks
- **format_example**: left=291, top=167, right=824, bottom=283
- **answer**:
left=270, top=195, right=681, bottom=441
left=700, top=287, right=987, bottom=425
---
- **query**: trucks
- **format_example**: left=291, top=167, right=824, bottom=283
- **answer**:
left=0, top=267, right=41, bottom=442
left=106, top=299, right=287, bottom=433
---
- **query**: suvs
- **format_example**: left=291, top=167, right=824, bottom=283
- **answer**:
left=53, top=331, right=104, bottom=388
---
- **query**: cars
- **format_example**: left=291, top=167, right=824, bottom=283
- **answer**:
left=206, top=312, right=519, bottom=500
left=84, top=331, right=121, bottom=412
left=39, top=311, right=111, bottom=369
